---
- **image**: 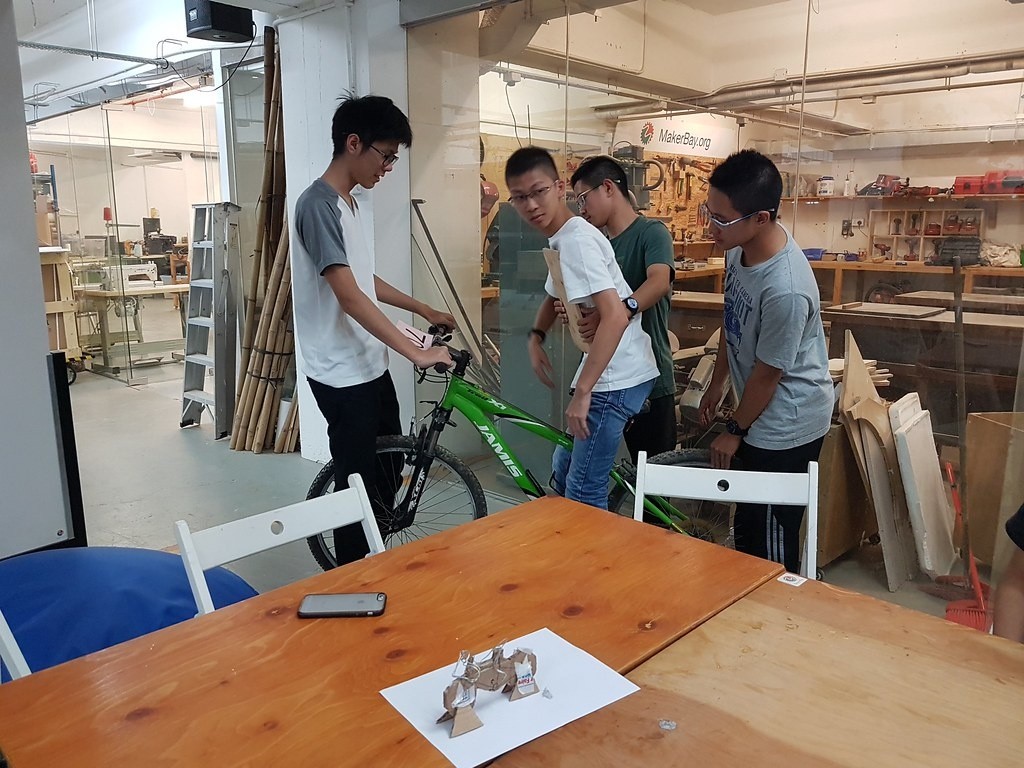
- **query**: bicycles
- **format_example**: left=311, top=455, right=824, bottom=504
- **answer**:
left=305, top=322, right=744, bottom=572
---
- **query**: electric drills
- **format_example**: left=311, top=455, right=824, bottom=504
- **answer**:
left=874, top=244, right=891, bottom=260
left=924, top=240, right=944, bottom=266
left=891, top=218, right=902, bottom=235
left=903, top=239, right=918, bottom=261
left=907, top=214, right=919, bottom=235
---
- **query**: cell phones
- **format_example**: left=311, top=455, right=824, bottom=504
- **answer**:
left=297, top=592, right=387, bottom=619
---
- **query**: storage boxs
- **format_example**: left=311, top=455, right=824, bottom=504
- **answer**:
left=953, top=176, right=985, bottom=194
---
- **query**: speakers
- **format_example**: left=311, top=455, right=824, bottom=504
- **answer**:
left=184, top=0, right=254, bottom=44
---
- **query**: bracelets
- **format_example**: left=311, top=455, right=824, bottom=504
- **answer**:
left=528, top=329, right=546, bottom=343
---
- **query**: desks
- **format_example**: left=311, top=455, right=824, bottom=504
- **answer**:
left=0, top=492, right=1024, bottom=768
left=74, top=284, right=190, bottom=375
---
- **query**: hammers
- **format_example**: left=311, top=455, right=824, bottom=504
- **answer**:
left=686, top=172, right=695, bottom=200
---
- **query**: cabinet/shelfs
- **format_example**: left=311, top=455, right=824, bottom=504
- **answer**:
left=669, top=193, right=1024, bottom=484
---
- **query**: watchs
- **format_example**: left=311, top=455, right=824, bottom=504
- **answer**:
left=726, top=417, right=749, bottom=435
left=622, top=297, right=639, bottom=317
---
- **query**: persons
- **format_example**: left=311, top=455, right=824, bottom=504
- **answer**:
left=699, top=150, right=835, bottom=573
left=992, top=503, right=1024, bottom=643
left=291, top=96, right=455, bottom=568
left=506, top=146, right=659, bottom=511
left=528, top=155, right=676, bottom=528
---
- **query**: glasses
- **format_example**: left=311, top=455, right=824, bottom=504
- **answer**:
left=700, top=200, right=774, bottom=231
left=343, top=133, right=400, bottom=166
left=576, top=179, right=621, bottom=210
left=507, top=180, right=559, bottom=208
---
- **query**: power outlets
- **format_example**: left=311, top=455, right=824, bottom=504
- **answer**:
left=851, top=218, right=864, bottom=227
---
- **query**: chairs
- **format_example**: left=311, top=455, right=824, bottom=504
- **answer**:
left=634, top=449, right=821, bottom=581
left=176, top=472, right=389, bottom=625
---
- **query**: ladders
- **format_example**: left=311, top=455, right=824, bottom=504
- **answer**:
left=179, top=202, right=244, bottom=440
